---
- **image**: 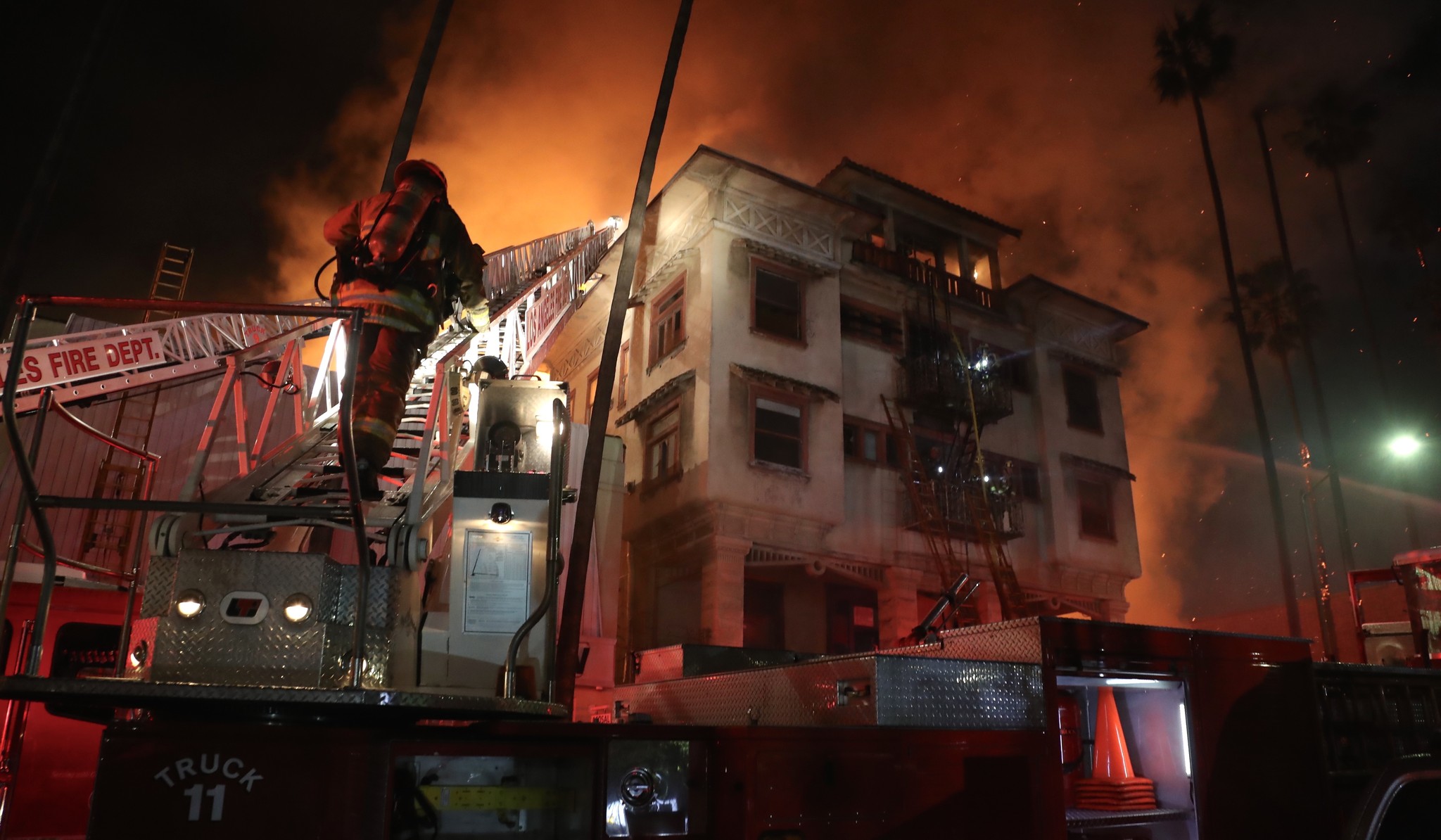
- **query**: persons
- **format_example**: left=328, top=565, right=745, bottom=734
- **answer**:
left=325, top=160, right=487, bottom=502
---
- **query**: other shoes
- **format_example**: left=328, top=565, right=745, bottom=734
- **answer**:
left=355, top=458, right=385, bottom=501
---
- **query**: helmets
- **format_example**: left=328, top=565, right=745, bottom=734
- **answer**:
left=394, top=159, right=447, bottom=193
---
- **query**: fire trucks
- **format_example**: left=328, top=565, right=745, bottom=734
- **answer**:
left=413, top=546, right=1441, bottom=840
left=0, top=292, right=732, bottom=840
